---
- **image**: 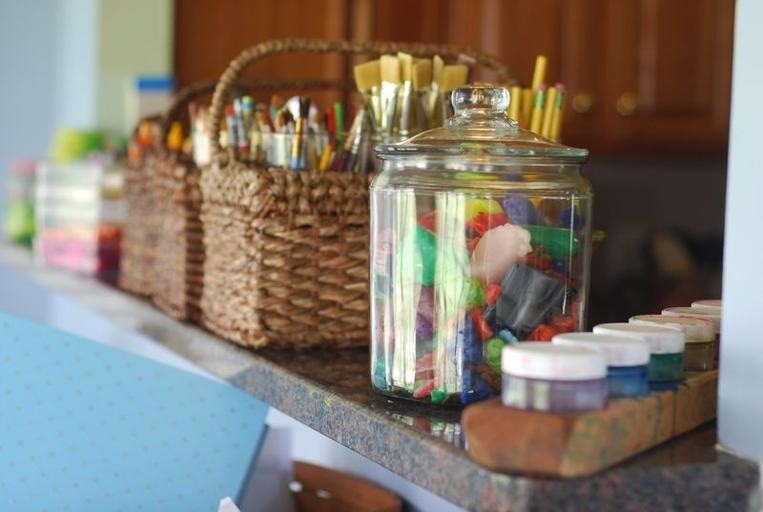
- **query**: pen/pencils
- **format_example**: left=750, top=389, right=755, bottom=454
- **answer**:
left=531, top=54, right=547, bottom=90
left=217, top=93, right=324, bottom=175
left=521, top=89, right=530, bottom=127
left=369, top=81, right=451, bottom=131
left=334, top=101, right=343, bottom=158
left=325, top=105, right=335, bottom=134
left=348, top=123, right=360, bottom=172
left=530, top=85, right=543, bottom=134
left=549, top=91, right=563, bottom=141
left=556, top=83, right=565, bottom=91
left=542, top=87, right=556, bottom=139
left=338, top=106, right=358, bottom=169
left=360, top=131, right=371, bottom=173
left=509, top=86, right=520, bottom=121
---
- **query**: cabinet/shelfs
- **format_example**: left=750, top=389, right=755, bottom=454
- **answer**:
left=173, top=0, right=734, bottom=167
